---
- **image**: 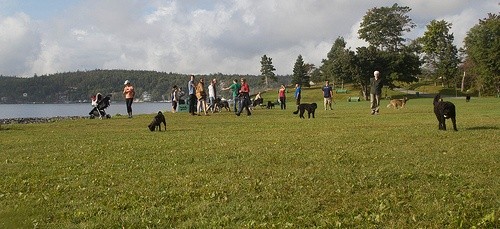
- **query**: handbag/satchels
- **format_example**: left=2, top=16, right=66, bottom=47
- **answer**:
left=199, top=89, right=206, bottom=101
left=241, top=92, right=251, bottom=105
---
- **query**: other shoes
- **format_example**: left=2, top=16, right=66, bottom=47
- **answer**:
left=375, top=112, right=378, bottom=115
left=371, top=111, right=375, bottom=115
left=191, top=113, right=197, bottom=115
left=128, top=115, right=132, bottom=118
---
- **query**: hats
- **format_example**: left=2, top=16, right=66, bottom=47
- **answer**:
left=124, top=80, right=130, bottom=85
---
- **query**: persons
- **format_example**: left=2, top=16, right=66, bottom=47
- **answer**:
left=294, top=84, right=301, bottom=110
left=254, top=91, right=263, bottom=106
left=278, top=84, right=287, bottom=110
left=370, top=71, right=383, bottom=116
left=123, top=80, right=135, bottom=118
left=170, top=74, right=251, bottom=116
left=322, top=79, right=333, bottom=110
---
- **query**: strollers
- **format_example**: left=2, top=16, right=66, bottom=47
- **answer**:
left=88, top=92, right=112, bottom=120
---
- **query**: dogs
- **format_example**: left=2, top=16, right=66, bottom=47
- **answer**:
left=433, top=97, right=459, bottom=132
left=267, top=101, right=276, bottom=109
left=387, top=96, right=408, bottom=110
left=465, top=94, right=470, bottom=102
left=248, top=97, right=263, bottom=110
left=213, top=97, right=231, bottom=112
left=293, top=103, right=317, bottom=119
left=148, top=111, right=166, bottom=131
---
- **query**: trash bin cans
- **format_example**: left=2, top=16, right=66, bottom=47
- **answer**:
left=416, top=92, right=419, bottom=96
